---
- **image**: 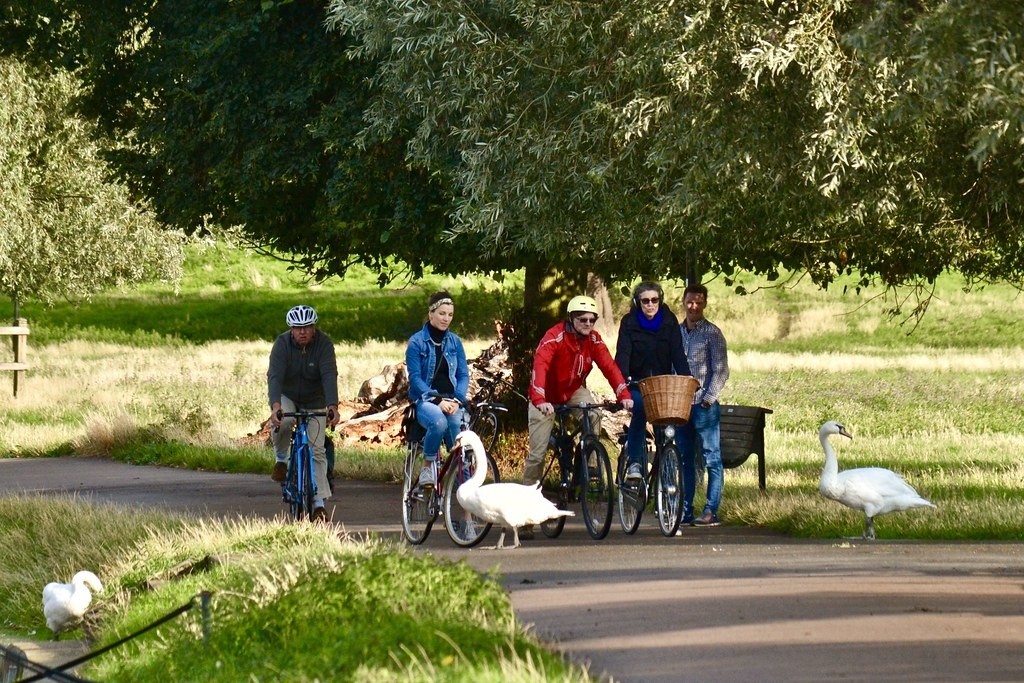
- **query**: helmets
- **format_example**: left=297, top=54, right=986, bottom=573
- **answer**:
left=286, top=305, right=318, bottom=327
left=567, top=295, right=598, bottom=317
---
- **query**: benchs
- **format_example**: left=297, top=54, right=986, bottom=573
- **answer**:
left=615, top=405, right=773, bottom=493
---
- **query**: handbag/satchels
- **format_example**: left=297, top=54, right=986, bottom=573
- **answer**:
left=403, top=403, right=427, bottom=441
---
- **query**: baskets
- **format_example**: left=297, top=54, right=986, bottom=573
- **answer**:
left=637, top=375, right=700, bottom=428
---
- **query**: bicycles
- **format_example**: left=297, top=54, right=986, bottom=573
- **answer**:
left=614, top=375, right=705, bottom=537
left=274, top=409, right=335, bottom=522
left=401, top=392, right=509, bottom=548
left=539, top=398, right=625, bottom=540
left=467, top=363, right=529, bottom=456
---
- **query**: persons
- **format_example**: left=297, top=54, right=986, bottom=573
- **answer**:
left=519, top=296, right=633, bottom=539
left=405, top=292, right=477, bottom=542
left=673, top=283, right=730, bottom=526
left=613, top=282, right=699, bottom=533
left=267, top=305, right=340, bottom=528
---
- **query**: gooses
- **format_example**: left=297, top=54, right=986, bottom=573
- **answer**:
left=817, top=420, right=938, bottom=539
left=41, top=570, right=104, bottom=632
left=450, top=430, right=576, bottom=550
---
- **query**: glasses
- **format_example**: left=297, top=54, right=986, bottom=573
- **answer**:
left=639, top=297, right=661, bottom=304
left=572, top=313, right=597, bottom=324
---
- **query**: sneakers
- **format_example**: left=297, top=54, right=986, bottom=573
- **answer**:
left=664, top=476, right=676, bottom=493
left=419, top=468, right=434, bottom=486
left=518, top=525, right=535, bottom=540
left=464, top=519, right=477, bottom=539
left=589, top=467, right=599, bottom=478
left=663, top=515, right=695, bottom=526
left=313, top=507, right=328, bottom=522
left=691, top=512, right=721, bottom=527
left=628, top=462, right=642, bottom=478
left=271, top=462, right=287, bottom=481
